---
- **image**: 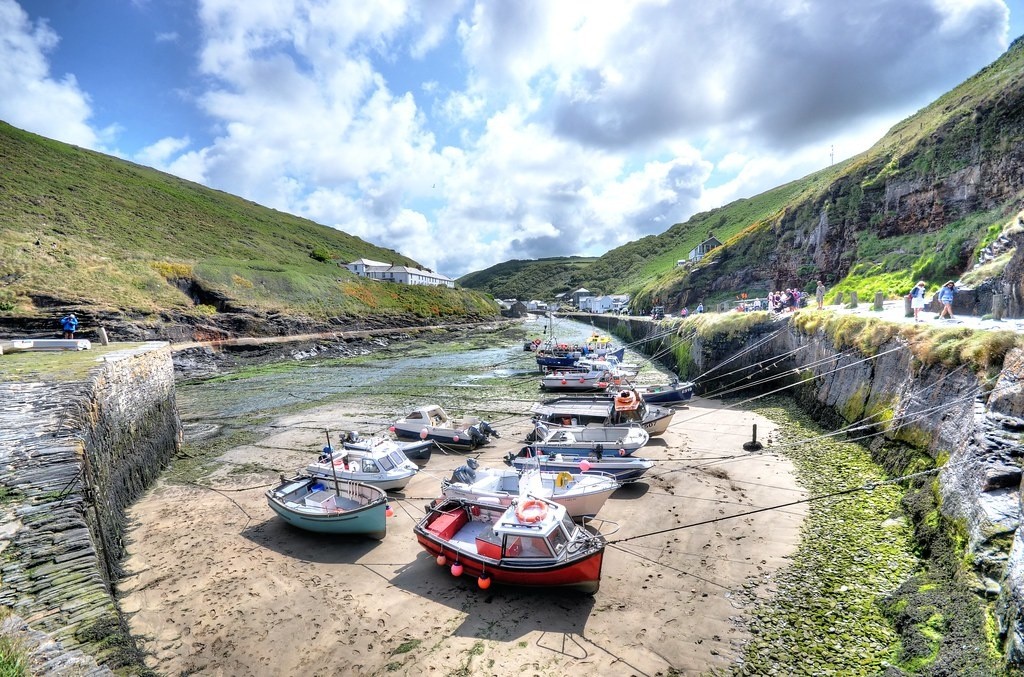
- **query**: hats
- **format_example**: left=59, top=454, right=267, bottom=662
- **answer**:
left=70, top=314, right=75, bottom=319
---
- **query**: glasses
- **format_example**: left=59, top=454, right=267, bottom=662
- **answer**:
left=948, top=284, right=953, bottom=287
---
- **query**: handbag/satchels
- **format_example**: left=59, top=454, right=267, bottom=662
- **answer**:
left=908, top=294, right=914, bottom=302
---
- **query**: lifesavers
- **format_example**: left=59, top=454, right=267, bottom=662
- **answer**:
left=557, top=473, right=573, bottom=488
left=516, top=499, right=548, bottom=524
left=618, top=390, right=634, bottom=403
left=604, top=372, right=613, bottom=381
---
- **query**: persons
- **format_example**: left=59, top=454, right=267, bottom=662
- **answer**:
left=910, top=280, right=959, bottom=321
left=767, top=288, right=801, bottom=313
left=697, top=303, right=703, bottom=313
left=754, top=298, right=762, bottom=311
left=593, top=444, right=603, bottom=462
left=681, top=307, right=689, bottom=318
left=815, top=281, right=825, bottom=311
left=60, top=314, right=78, bottom=339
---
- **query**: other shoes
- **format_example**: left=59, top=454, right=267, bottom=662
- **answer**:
left=939, top=315, right=945, bottom=320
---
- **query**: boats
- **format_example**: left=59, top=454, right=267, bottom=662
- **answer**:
left=264, top=469, right=387, bottom=541
left=305, top=433, right=419, bottom=492
left=412, top=495, right=604, bottom=595
left=530, top=386, right=676, bottom=437
left=440, top=457, right=622, bottom=525
left=504, top=417, right=650, bottom=458
left=392, top=404, right=503, bottom=451
left=504, top=453, right=655, bottom=483
left=339, top=430, right=435, bottom=465
left=534, top=327, right=696, bottom=405
